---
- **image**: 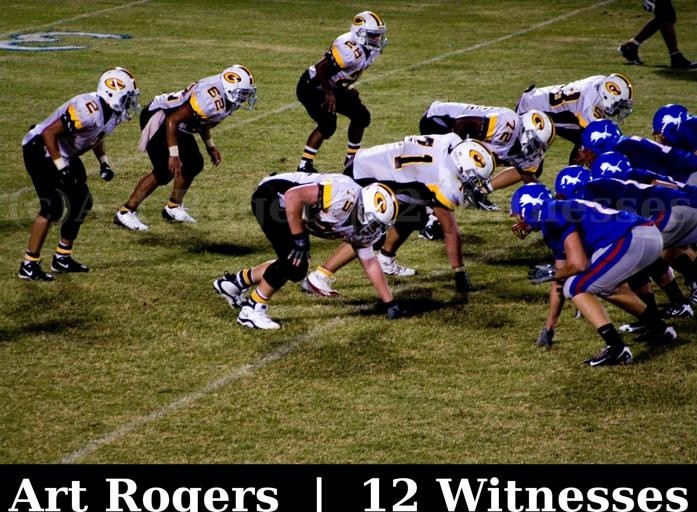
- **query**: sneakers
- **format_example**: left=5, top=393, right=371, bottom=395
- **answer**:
left=18, top=257, right=54, bottom=280
left=671, top=54, right=697, bottom=69
left=617, top=42, right=644, bottom=65
left=113, top=207, right=149, bottom=231
left=213, top=252, right=416, bottom=330
left=51, top=253, right=89, bottom=273
left=583, top=287, right=697, bottom=368
left=162, top=202, right=197, bottom=224
left=297, top=163, right=318, bottom=173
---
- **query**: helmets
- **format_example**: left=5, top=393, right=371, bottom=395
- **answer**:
left=97, top=67, right=141, bottom=121
left=349, top=10, right=387, bottom=57
left=357, top=73, right=694, bottom=247
left=221, top=64, right=258, bottom=111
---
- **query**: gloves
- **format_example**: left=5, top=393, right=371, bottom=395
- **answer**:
left=58, top=164, right=77, bottom=186
left=100, top=161, right=114, bottom=182
left=537, top=327, right=554, bottom=347
left=453, top=271, right=470, bottom=293
left=528, top=263, right=555, bottom=285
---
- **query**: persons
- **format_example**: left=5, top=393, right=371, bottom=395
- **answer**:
left=113, top=64, right=257, bottom=232
left=467, top=73, right=634, bottom=211
left=617, top=0, right=696, bottom=70
left=17, top=66, right=140, bottom=281
left=512, top=103, right=696, bottom=365
left=212, top=171, right=407, bottom=330
left=301, top=131, right=496, bottom=299
left=418, top=99, right=555, bottom=240
left=296, top=9, right=388, bottom=174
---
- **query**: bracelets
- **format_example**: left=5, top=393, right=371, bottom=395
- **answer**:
left=53, top=157, right=67, bottom=171
left=99, top=154, right=108, bottom=165
left=452, top=264, right=466, bottom=274
left=203, top=137, right=215, bottom=149
left=169, top=144, right=180, bottom=158
